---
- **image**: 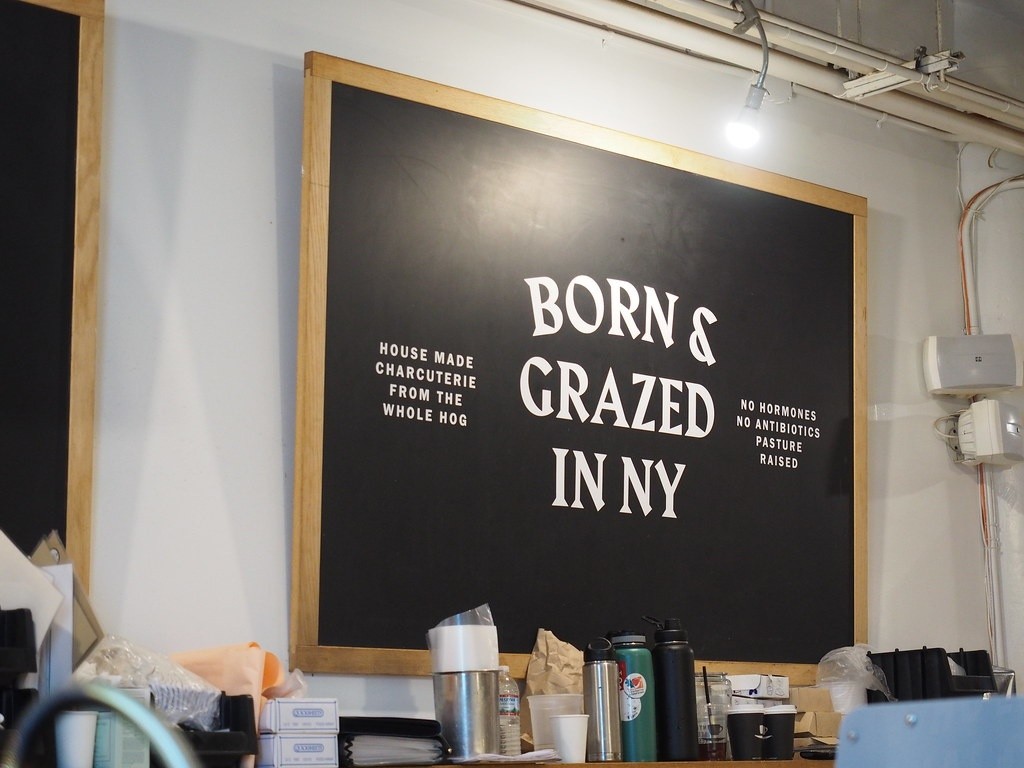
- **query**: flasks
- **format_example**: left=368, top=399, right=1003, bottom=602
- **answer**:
left=611, top=631, right=656, bottom=763
left=584, top=635, right=625, bottom=762
left=644, top=613, right=700, bottom=762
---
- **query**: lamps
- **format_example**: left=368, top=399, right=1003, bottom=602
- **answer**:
left=728, top=1, right=775, bottom=136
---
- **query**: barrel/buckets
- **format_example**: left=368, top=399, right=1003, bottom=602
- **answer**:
left=430, top=668, right=498, bottom=757
left=526, top=690, right=584, bottom=759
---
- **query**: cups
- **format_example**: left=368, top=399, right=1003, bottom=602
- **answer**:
left=764, top=705, right=797, bottom=760
left=54, top=708, right=100, bottom=768
left=546, top=712, right=589, bottom=763
left=693, top=671, right=732, bottom=761
left=728, top=703, right=765, bottom=761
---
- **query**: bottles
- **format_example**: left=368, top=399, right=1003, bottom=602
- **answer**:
left=496, top=663, right=522, bottom=755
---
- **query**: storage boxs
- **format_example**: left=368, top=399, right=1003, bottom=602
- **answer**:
left=254, top=731, right=339, bottom=767
left=256, top=696, right=343, bottom=734
